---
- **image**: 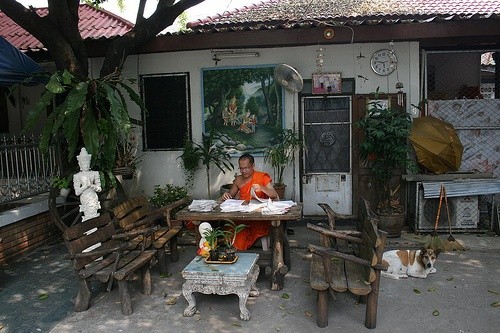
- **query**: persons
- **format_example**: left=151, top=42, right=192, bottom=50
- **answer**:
left=220, top=152, right=280, bottom=252
left=74, top=147, right=104, bottom=262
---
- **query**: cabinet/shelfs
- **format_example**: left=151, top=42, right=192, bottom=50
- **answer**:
left=351, top=92, right=408, bottom=220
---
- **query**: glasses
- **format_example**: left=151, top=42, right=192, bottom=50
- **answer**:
left=238, top=165, right=252, bottom=170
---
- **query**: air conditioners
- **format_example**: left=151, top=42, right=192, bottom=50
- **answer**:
left=418, top=189, right=477, bottom=230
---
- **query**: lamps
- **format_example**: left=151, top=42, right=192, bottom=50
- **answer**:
left=209, top=48, right=259, bottom=59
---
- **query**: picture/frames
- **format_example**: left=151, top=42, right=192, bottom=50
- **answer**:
left=138, top=72, right=192, bottom=153
left=311, top=71, right=341, bottom=95
left=199, top=65, right=286, bottom=158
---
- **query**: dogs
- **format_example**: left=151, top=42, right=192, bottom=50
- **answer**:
left=380, top=248, right=441, bottom=279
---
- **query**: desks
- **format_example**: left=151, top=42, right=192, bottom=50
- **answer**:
left=181, top=254, right=262, bottom=320
left=175, top=200, right=302, bottom=292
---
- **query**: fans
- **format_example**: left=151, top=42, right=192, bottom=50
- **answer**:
left=272, top=62, right=304, bottom=95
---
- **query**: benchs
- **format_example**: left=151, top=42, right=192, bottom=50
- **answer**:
left=306, top=197, right=389, bottom=329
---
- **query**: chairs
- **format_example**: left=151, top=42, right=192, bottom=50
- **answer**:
left=64, top=195, right=182, bottom=316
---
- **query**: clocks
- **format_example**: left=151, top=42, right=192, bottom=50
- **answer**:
left=371, top=49, right=398, bottom=76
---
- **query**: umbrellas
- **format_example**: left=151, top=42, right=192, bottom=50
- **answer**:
left=408, top=114, right=464, bottom=174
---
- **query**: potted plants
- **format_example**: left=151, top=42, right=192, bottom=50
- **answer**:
left=201, top=218, right=246, bottom=262
left=263, top=128, right=305, bottom=201
left=356, top=104, right=410, bottom=237
